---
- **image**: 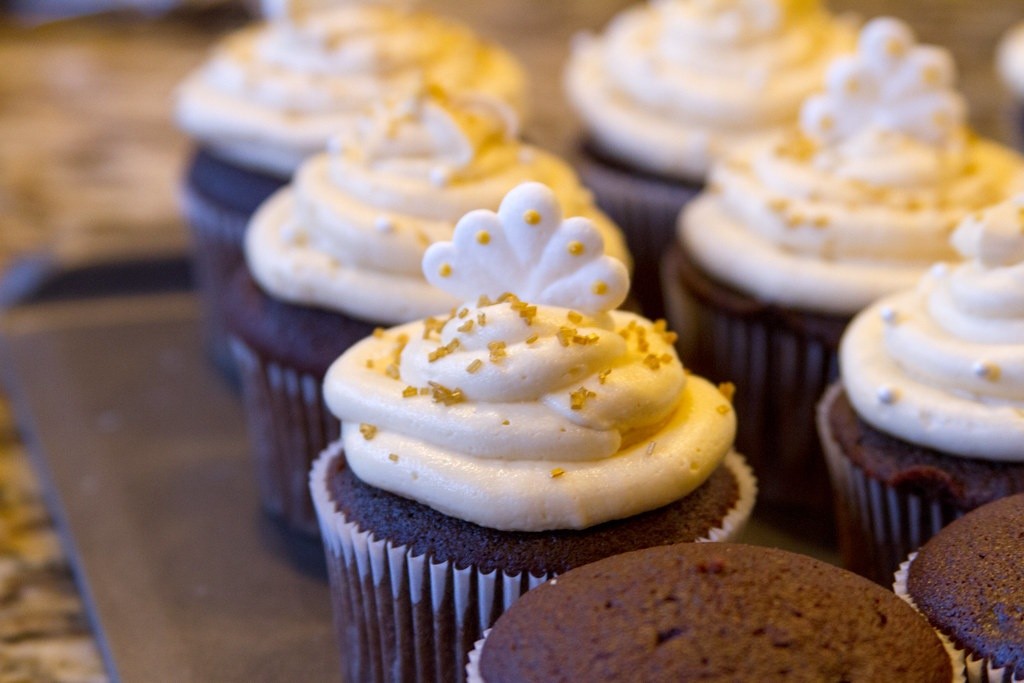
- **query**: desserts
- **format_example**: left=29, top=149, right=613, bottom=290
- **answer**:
left=173, top=0, right=1024, bottom=683
